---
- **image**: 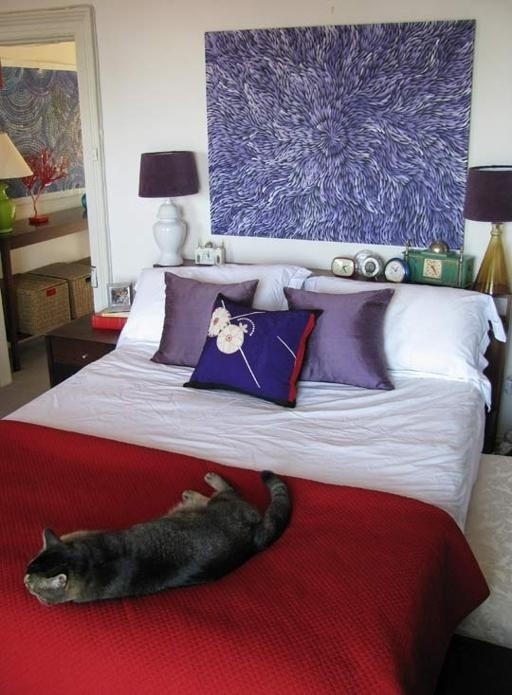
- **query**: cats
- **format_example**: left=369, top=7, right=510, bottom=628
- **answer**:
left=22, top=470, right=292, bottom=607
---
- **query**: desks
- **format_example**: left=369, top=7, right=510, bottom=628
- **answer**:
left=0, top=201, right=97, bottom=375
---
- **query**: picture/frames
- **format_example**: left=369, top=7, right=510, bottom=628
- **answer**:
left=0, top=59, right=87, bottom=206
left=105, top=281, right=135, bottom=314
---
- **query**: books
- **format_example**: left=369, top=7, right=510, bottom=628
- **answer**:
left=91, top=307, right=131, bottom=331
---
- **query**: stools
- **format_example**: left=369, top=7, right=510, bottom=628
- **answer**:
left=446, top=440, right=512, bottom=694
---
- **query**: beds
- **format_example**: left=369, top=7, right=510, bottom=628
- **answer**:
left=2, top=260, right=508, bottom=694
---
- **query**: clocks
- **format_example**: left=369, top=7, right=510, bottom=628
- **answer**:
left=359, top=254, right=386, bottom=280
left=400, top=240, right=476, bottom=289
left=383, top=257, right=412, bottom=286
left=355, top=249, right=374, bottom=272
left=331, top=256, right=357, bottom=279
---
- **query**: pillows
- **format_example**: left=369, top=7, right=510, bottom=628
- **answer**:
left=148, top=266, right=259, bottom=373
left=280, top=284, right=396, bottom=395
left=179, top=290, right=326, bottom=408
left=303, top=268, right=507, bottom=413
left=116, top=261, right=310, bottom=365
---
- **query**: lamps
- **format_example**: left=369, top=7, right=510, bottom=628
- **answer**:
left=461, top=161, right=512, bottom=299
left=131, top=144, right=201, bottom=266
left=0, top=125, right=32, bottom=233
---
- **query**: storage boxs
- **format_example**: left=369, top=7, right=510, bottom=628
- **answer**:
left=26, top=262, right=95, bottom=320
left=3, top=270, right=73, bottom=338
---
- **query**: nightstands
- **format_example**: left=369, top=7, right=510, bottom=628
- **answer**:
left=43, top=313, right=126, bottom=387
